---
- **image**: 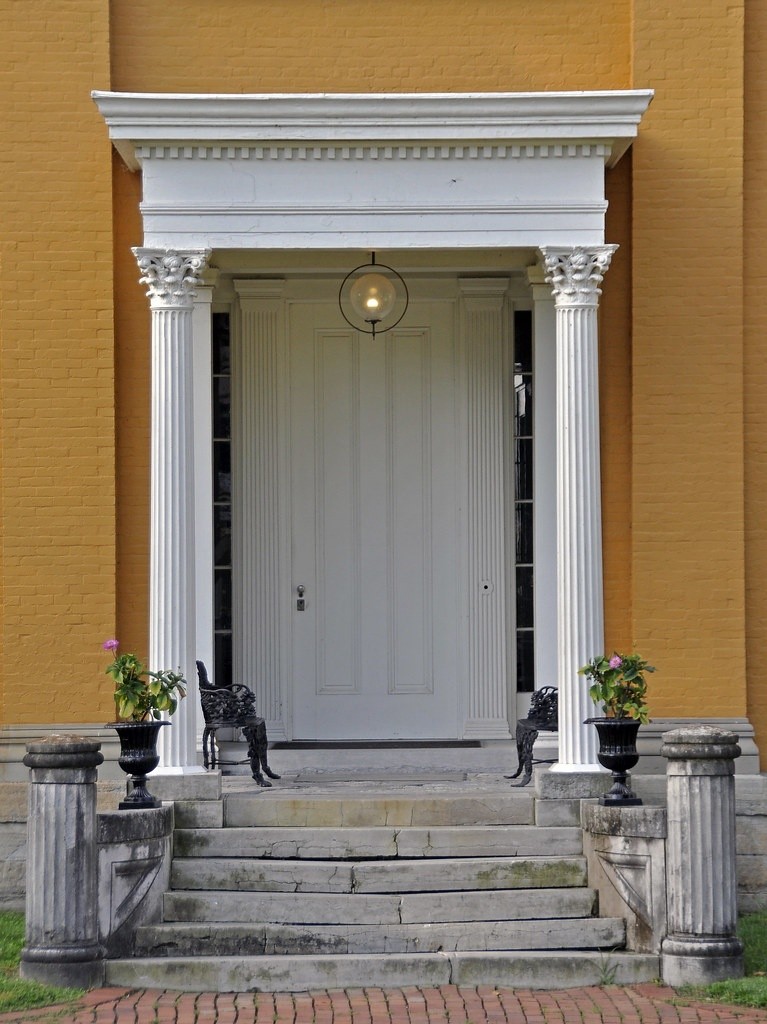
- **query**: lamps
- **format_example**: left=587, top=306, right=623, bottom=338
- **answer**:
left=339, top=251, right=408, bottom=341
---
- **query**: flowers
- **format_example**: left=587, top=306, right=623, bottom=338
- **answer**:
left=579, top=650, right=659, bottom=726
left=100, top=639, right=189, bottom=724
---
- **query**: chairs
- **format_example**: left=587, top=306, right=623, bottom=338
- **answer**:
left=195, top=660, right=283, bottom=788
left=503, top=685, right=559, bottom=787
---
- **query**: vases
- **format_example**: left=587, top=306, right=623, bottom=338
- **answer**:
left=582, top=715, right=646, bottom=806
left=103, top=720, right=173, bottom=810
